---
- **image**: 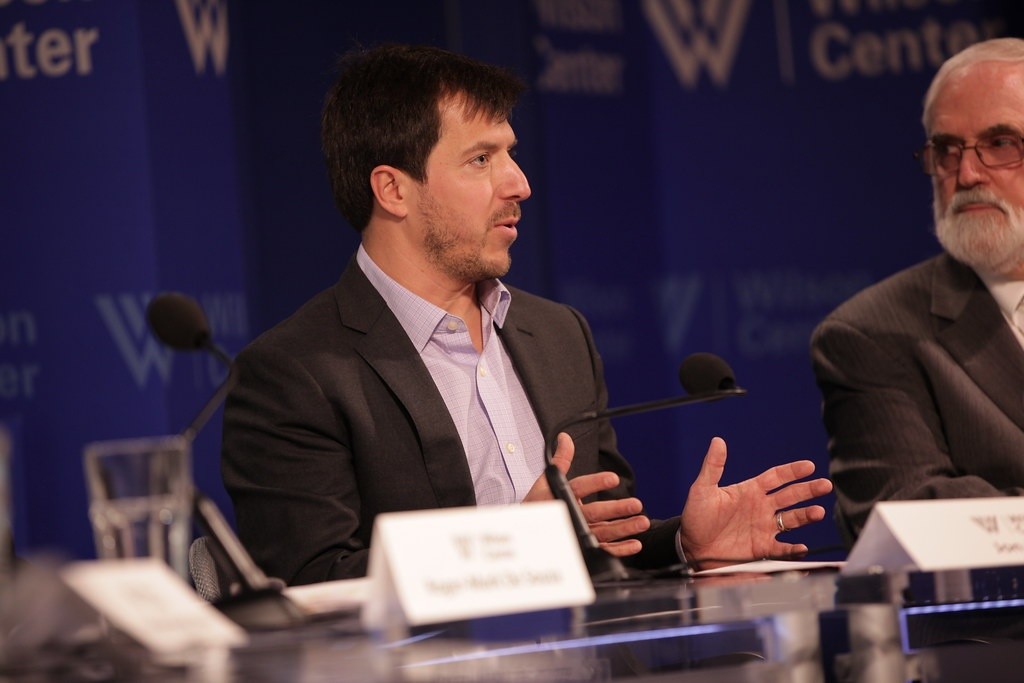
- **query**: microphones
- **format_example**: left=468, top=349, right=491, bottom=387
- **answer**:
left=142, top=289, right=307, bottom=629
left=542, top=350, right=750, bottom=582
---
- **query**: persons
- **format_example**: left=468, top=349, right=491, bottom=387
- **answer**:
left=220, top=42, right=834, bottom=587
left=811, top=38, right=1024, bottom=557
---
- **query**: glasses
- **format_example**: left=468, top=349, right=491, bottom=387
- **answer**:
left=913, top=132, right=1024, bottom=175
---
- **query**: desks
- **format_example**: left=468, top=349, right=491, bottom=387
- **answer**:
left=0, top=562, right=1024, bottom=683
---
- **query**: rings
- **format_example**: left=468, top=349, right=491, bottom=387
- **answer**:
left=777, top=512, right=791, bottom=532
